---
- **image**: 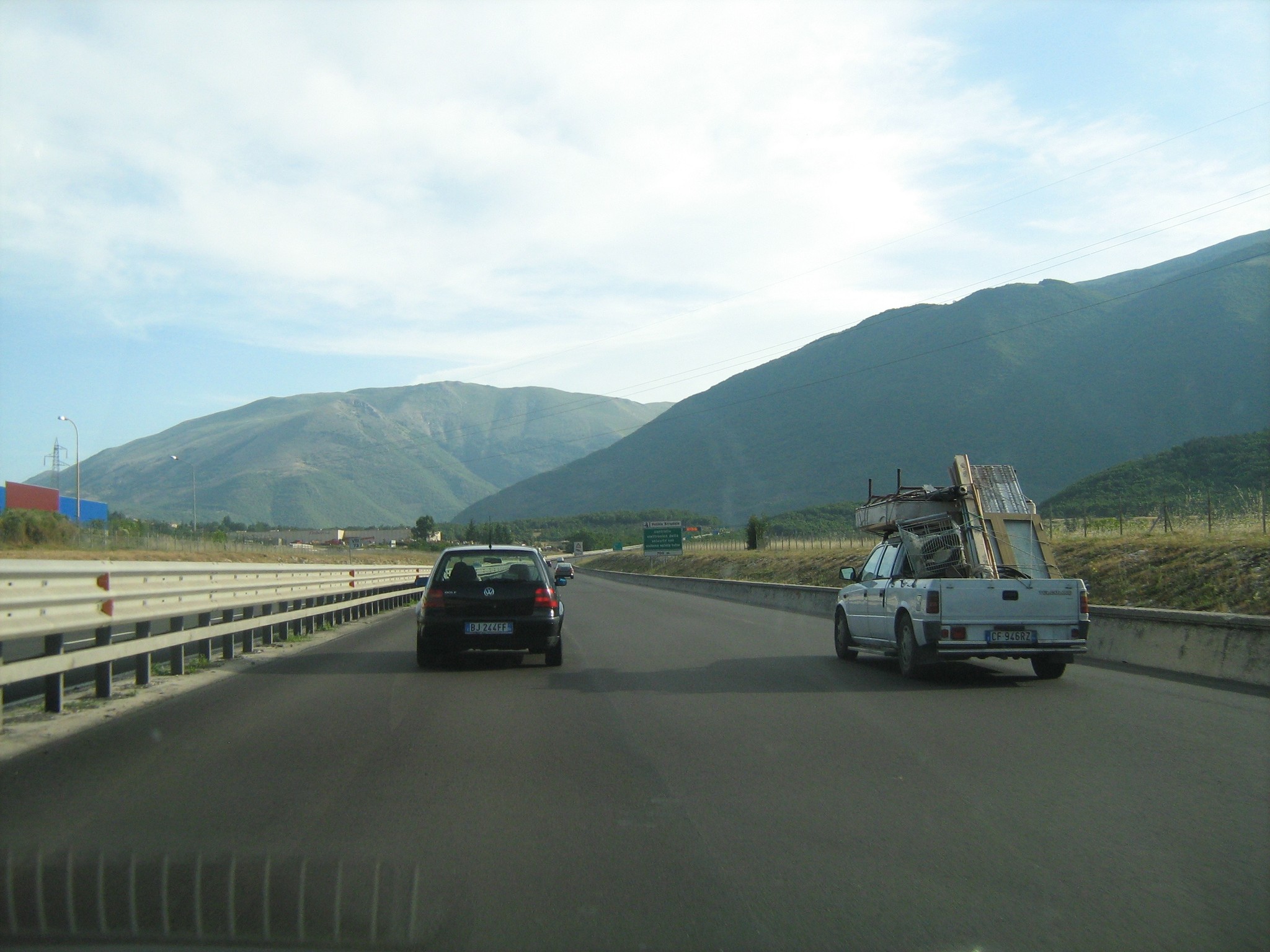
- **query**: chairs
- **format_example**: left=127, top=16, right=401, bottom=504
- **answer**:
left=450, top=562, right=482, bottom=582
left=508, top=564, right=534, bottom=580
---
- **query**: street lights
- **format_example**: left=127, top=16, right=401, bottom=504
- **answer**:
left=170, top=455, right=196, bottom=535
left=58, top=415, right=81, bottom=541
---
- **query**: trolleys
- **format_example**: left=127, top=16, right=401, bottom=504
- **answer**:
left=894, top=510, right=970, bottom=579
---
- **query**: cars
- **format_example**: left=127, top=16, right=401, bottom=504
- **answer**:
left=546, top=559, right=552, bottom=568
left=553, top=562, right=574, bottom=579
left=556, top=556, right=564, bottom=563
left=413, top=542, right=567, bottom=667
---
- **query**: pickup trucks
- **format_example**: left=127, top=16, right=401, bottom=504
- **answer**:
left=834, top=529, right=1092, bottom=680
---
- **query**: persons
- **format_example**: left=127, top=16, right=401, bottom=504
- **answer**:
left=454, top=562, right=482, bottom=581
